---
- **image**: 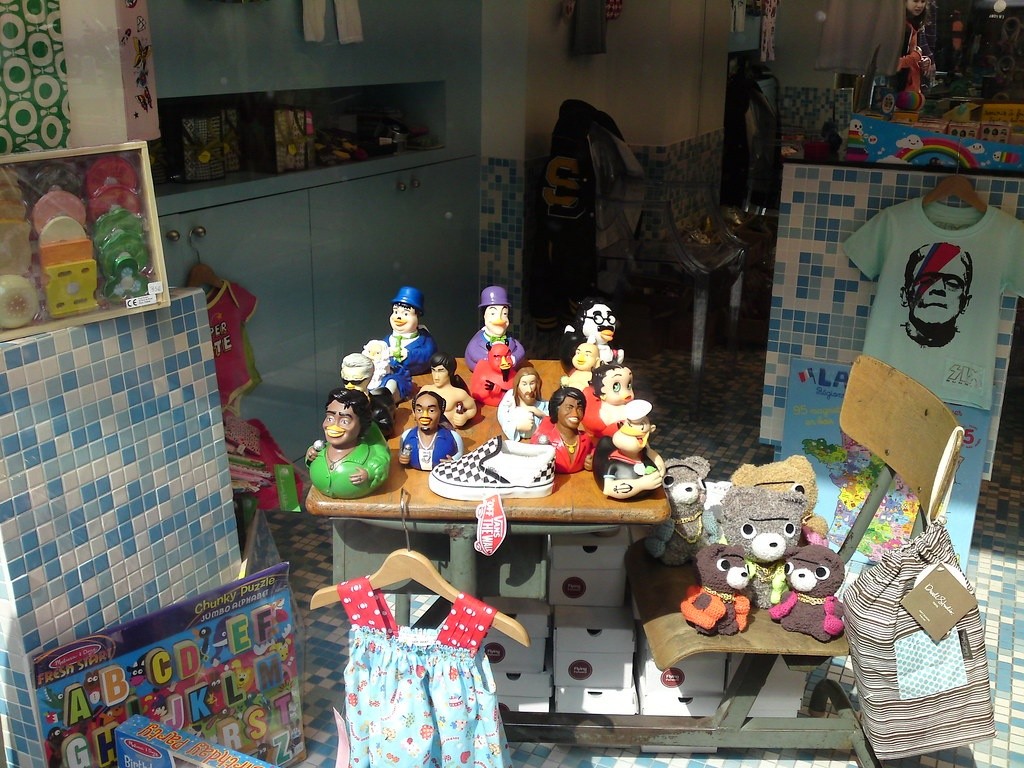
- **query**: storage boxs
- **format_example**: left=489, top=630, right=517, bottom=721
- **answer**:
left=858, top=107, right=1010, bottom=144
left=115, top=714, right=282, bottom=768
left=0, top=139, right=171, bottom=342
left=481, top=527, right=807, bottom=718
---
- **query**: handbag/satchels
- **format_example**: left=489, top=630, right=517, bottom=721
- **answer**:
left=181, top=104, right=243, bottom=182
left=843, top=425, right=997, bottom=759
left=246, top=103, right=316, bottom=172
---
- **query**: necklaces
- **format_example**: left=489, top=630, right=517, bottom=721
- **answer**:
left=327, top=447, right=351, bottom=470
left=417, top=431, right=438, bottom=460
left=564, top=440, right=577, bottom=453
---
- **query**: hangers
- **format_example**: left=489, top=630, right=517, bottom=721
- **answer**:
left=922, top=135, right=988, bottom=212
left=310, top=486, right=532, bottom=647
left=185, top=228, right=224, bottom=290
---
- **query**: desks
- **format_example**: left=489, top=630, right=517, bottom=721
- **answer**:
left=304, top=354, right=882, bottom=768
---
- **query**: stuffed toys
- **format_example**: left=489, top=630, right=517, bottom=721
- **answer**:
left=644, top=454, right=844, bottom=643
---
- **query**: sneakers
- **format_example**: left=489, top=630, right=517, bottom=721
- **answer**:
left=429, top=435, right=556, bottom=501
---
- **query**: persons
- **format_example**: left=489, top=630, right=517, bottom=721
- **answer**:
left=897, top=0, right=936, bottom=88
left=305, top=285, right=668, bottom=501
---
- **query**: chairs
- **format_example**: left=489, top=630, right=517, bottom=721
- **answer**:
left=624, top=354, right=963, bottom=732
left=586, top=124, right=750, bottom=424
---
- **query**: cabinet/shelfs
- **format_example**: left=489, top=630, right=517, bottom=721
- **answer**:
left=62, top=0, right=481, bottom=478
